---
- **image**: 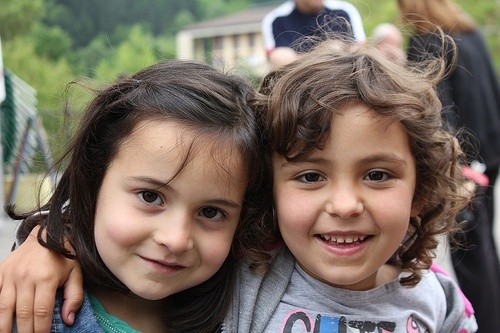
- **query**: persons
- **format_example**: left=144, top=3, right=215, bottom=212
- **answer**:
left=1, top=40, right=480, bottom=333
left=0, top=61, right=271, bottom=333
left=261, top=0, right=500, bottom=333
